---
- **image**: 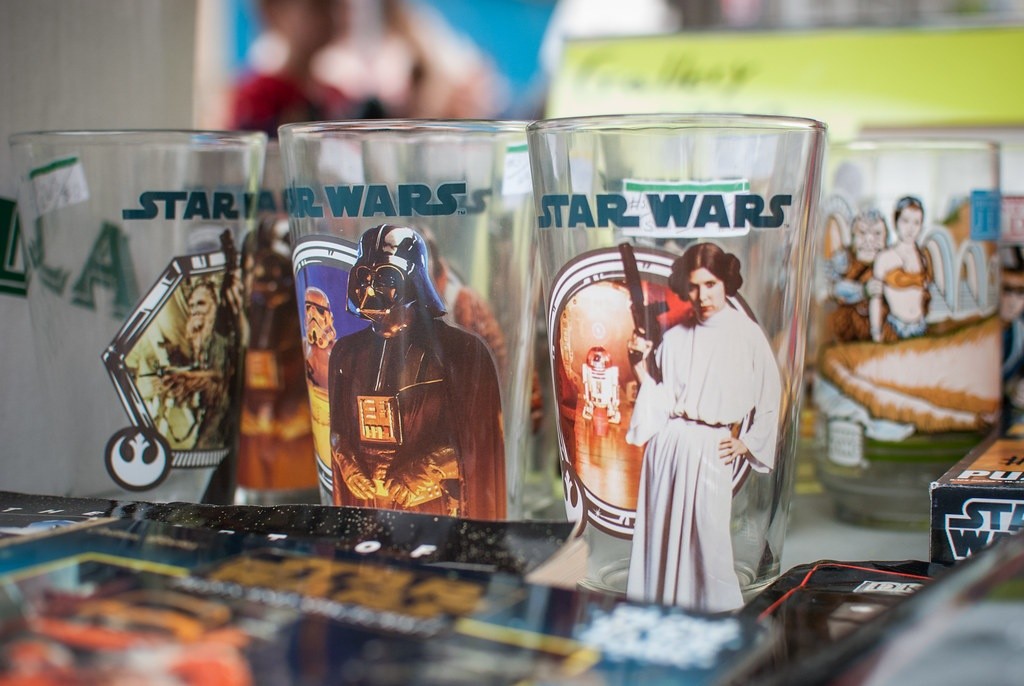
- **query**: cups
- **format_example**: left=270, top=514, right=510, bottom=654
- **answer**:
left=8, top=126, right=268, bottom=502
left=814, top=142, right=1002, bottom=523
left=276, top=122, right=540, bottom=519
left=528, top=114, right=825, bottom=594
